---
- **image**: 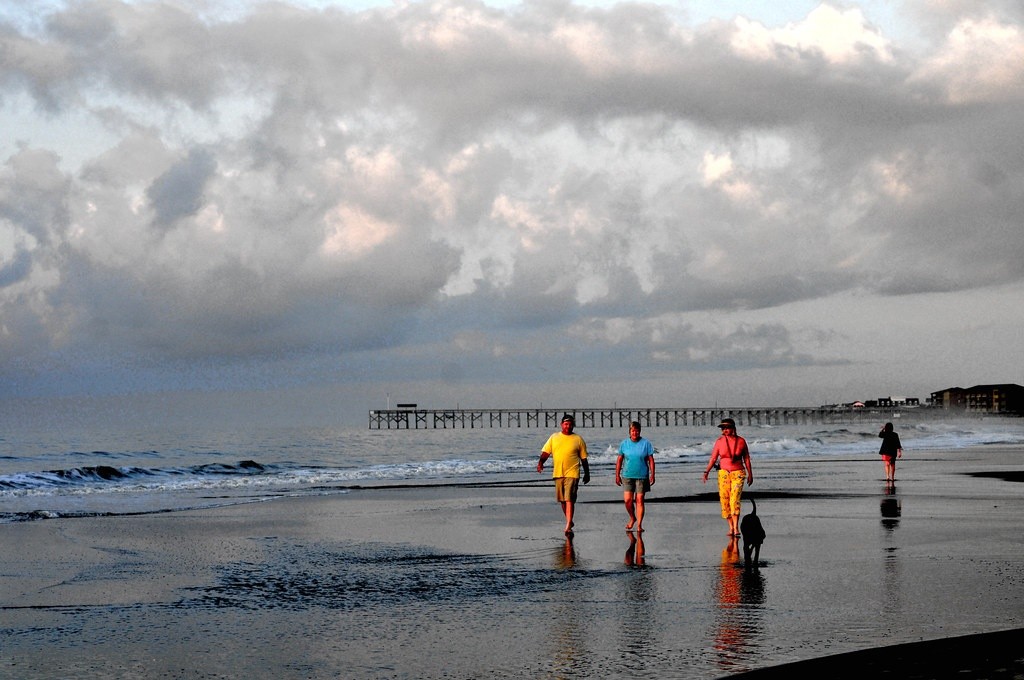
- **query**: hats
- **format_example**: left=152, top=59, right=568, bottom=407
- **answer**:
left=561, top=414, right=574, bottom=423
left=717, top=422, right=736, bottom=428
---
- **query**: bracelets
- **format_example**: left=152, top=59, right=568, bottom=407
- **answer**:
left=704, top=471, right=708, bottom=474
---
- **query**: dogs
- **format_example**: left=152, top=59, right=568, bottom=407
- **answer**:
left=740, top=492, right=767, bottom=563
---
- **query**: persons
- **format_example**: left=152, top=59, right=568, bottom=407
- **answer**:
left=878, top=422, right=902, bottom=480
left=616, top=421, right=656, bottom=532
left=703, top=418, right=753, bottom=536
left=536, top=414, right=591, bottom=535
